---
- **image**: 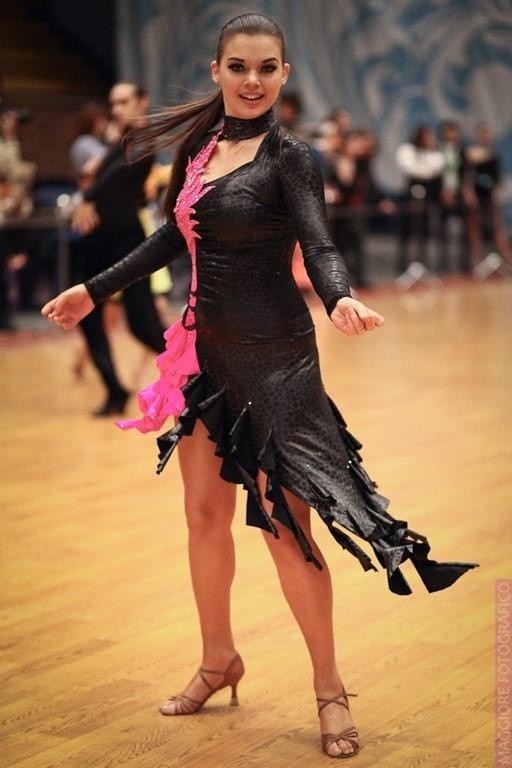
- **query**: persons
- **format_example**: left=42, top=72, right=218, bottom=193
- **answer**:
left=271, top=88, right=511, bottom=310
left=69, top=80, right=174, bottom=415
left=66, top=119, right=174, bottom=392
left=35, top=11, right=386, bottom=758
left=0, top=86, right=192, bottom=336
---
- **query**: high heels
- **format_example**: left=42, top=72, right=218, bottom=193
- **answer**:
left=159, top=652, right=246, bottom=716
left=90, top=386, right=130, bottom=416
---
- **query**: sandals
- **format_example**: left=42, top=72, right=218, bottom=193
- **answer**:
left=316, top=685, right=360, bottom=759
